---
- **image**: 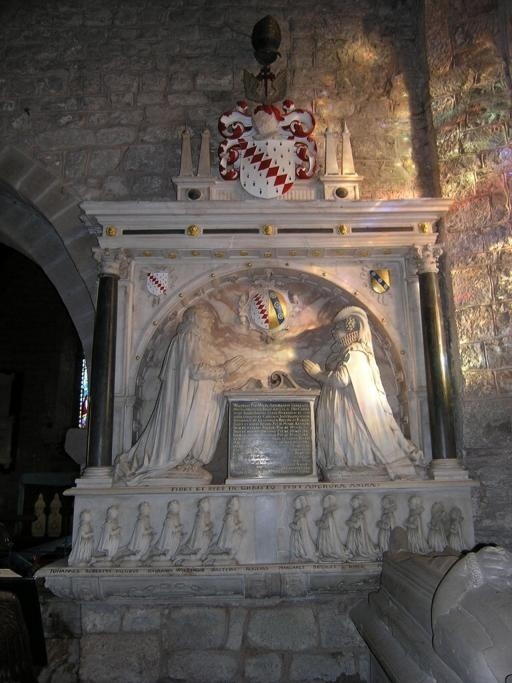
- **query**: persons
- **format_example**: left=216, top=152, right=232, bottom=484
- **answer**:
left=141, top=500, right=189, bottom=565
left=401, top=494, right=433, bottom=556
left=66, top=509, right=94, bottom=566
left=286, top=493, right=320, bottom=562
left=425, top=500, right=450, bottom=553
left=208, top=495, right=247, bottom=558
left=301, top=303, right=426, bottom=482
left=376, top=493, right=398, bottom=551
left=87, top=505, right=124, bottom=564
left=312, top=493, right=354, bottom=564
left=120, top=501, right=158, bottom=563
left=447, top=504, right=470, bottom=553
left=345, top=491, right=384, bottom=563
left=172, top=496, right=215, bottom=565
left=112, top=300, right=246, bottom=487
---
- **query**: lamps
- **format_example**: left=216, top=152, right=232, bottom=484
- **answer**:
left=216, top=13, right=320, bottom=200
